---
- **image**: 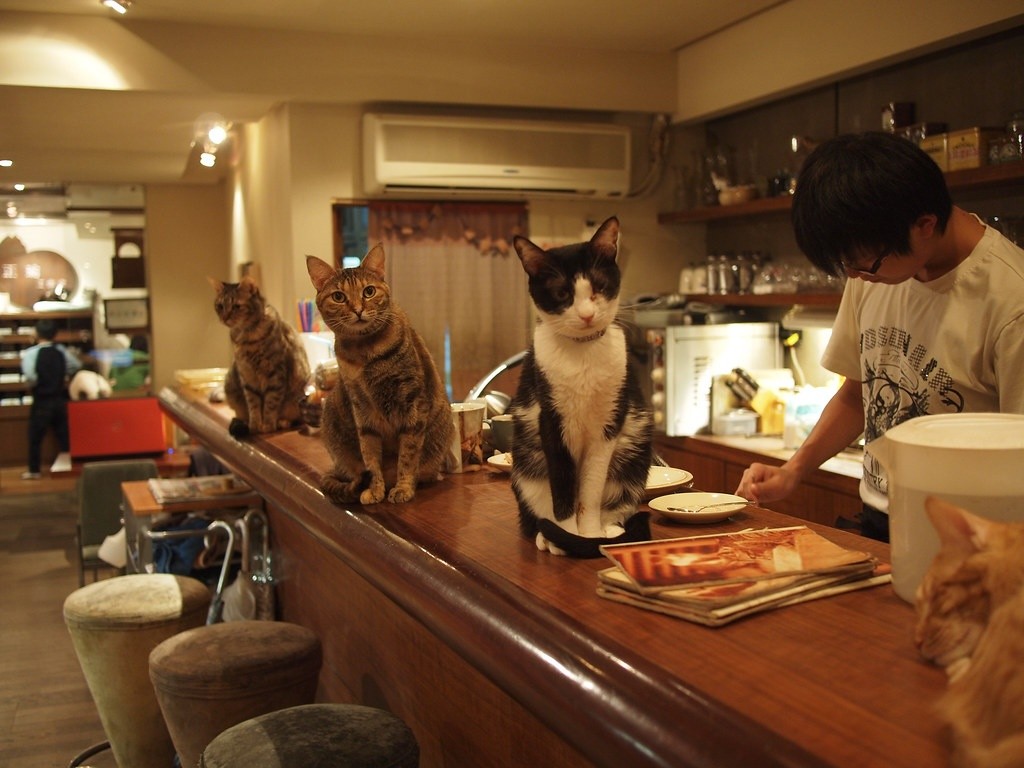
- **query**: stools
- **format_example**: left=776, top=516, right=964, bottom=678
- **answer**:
left=147, top=619, right=321, bottom=768
left=63, top=572, right=211, bottom=768
left=197, top=703, right=420, bottom=768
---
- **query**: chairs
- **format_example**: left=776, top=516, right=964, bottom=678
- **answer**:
left=72, top=459, right=158, bottom=586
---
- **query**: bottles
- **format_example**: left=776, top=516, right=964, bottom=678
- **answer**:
left=678, top=253, right=762, bottom=294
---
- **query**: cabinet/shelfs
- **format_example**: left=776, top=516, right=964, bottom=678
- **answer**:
left=649, top=441, right=864, bottom=538
left=654, top=97, right=1024, bottom=352
left=0, top=307, right=97, bottom=470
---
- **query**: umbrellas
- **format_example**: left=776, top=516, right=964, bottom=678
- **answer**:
left=205, top=511, right=274, bottom=625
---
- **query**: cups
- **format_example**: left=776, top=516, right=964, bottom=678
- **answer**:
left=753, top=263, right=848, bottom=295
left=439, top=402, right=486, bottom=474
left=482, top=414, right=514, bottom=453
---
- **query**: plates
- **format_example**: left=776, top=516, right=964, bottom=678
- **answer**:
left=648, top=492, right=748, bottom=524
left=646, top=465, right=695, bottom=499
left=487, top=452, right=513, bottom=473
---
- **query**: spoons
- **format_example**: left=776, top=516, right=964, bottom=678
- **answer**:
left=667, top=501, right=757, bottom=513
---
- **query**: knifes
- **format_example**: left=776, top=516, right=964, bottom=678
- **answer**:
left=726, top=368, right=758, bottom=401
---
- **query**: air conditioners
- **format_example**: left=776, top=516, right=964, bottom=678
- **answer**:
left=64, top=182, right=145, bottom=209
left=361, top=112, right=632, bottom=201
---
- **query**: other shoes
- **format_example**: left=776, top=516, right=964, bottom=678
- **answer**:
left=22, top=471, right=42, bottom=480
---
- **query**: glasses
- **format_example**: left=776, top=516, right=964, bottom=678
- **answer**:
left=831, top=223, right=913, bottom=277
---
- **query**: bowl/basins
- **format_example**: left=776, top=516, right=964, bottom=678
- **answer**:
left=711, top=412, right=759, bottom=437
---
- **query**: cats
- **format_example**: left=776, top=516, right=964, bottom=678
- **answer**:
left=513, top=214, right=653, bottom=555
left=304, top=242, right=454, bottom=504
left=915, top=497, right=1024, bottom=768
left=208, top=277, right=310, bottom=434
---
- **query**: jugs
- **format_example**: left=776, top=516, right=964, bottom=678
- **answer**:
left=862, top=412, right=1024, bottom=604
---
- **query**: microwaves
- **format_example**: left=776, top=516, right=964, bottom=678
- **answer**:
left=640, top=322, right=779, bottom=437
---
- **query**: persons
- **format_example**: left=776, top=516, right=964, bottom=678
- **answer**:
left=20, top=320, right=78, bottom=479
left=109, top=334, right=151, bottom=390
left=735, top=130, right=1024, bottom=543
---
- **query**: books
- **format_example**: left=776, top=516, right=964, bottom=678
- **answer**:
left=147, top=472, right=255, bottom=504
left=597, top=525, right=891, bottom=625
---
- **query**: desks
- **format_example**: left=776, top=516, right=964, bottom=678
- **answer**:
left=153, top=384, right=957, bottom=768
left=122, top=474, right=264, bottom=604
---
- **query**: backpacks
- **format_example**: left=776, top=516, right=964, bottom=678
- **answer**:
left=35, top=343, right=66, bottom=402
